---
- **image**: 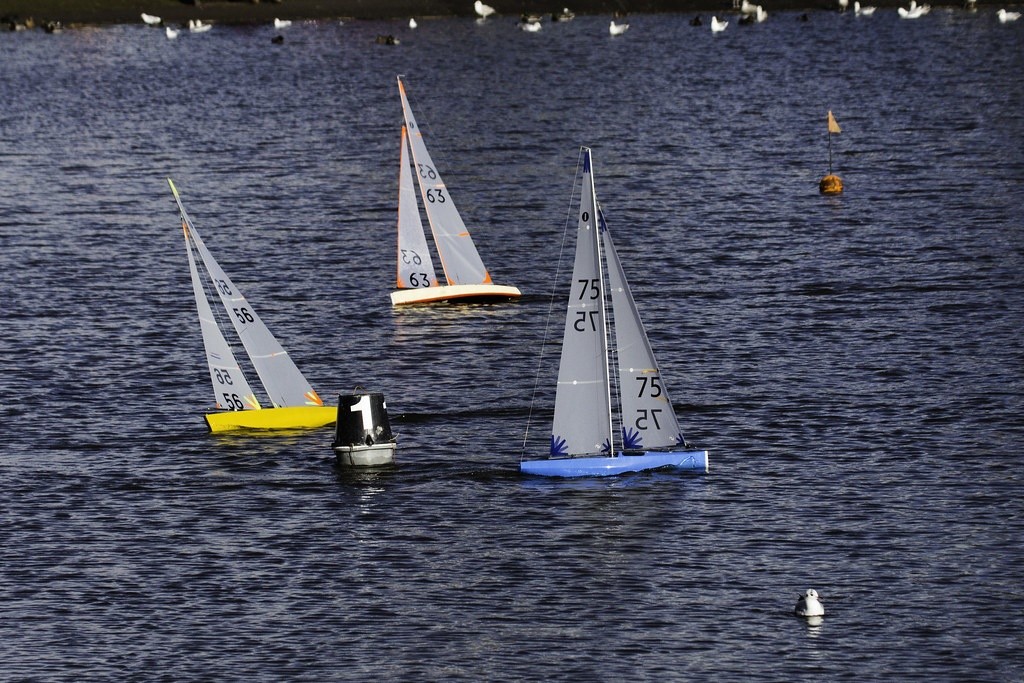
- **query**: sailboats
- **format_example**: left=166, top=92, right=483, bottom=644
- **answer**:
left=520, top=145, right=709, bottom=476
left=390, top=75, right=522, bottom=306
left=165, top=176, right=341, bottom=432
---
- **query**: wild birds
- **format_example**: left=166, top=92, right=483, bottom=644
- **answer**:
left=794, top=588, right=826, bottom=617
left=10, top=1, right=1024, bottom=47
